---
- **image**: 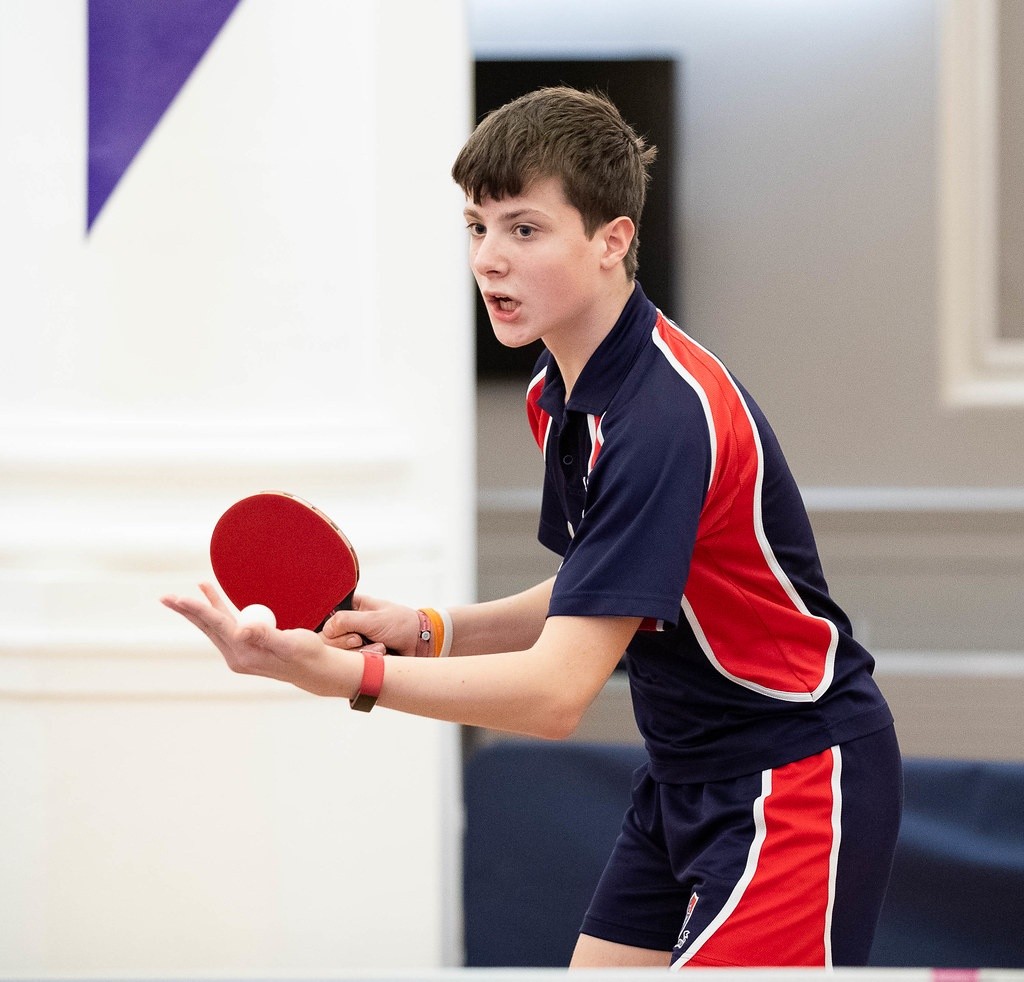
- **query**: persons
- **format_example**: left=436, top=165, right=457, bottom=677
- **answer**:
left=161, top=87, right=906, bottom=968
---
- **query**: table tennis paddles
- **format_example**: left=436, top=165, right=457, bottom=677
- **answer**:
left=209, top=493, right=398, bottom=662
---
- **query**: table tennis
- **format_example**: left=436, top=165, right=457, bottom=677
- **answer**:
left=236, top=602, right=276, bottom=631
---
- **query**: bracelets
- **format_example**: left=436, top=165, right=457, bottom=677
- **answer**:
left=414, top=605, right=453, bottom=658
left=348, top=648, right=385, bottom=713
